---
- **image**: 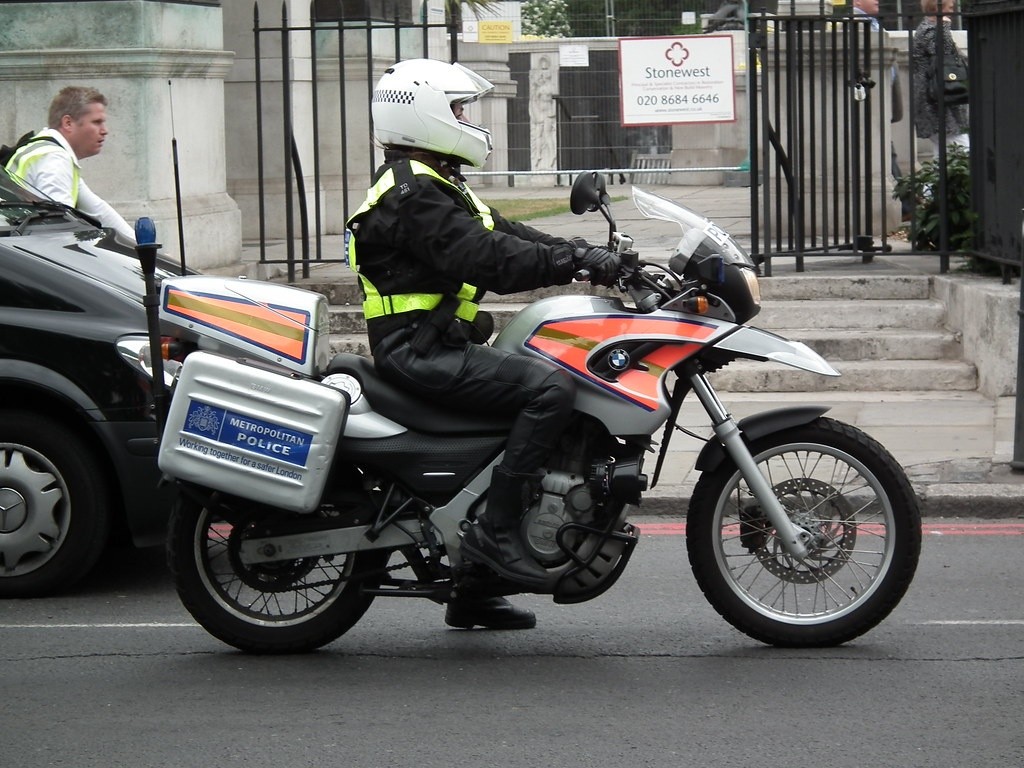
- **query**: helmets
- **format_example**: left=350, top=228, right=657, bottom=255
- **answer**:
left=370, top=57, right=496, bottom=168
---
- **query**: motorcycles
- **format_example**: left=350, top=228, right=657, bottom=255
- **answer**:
left=131, top=172, right=922, bottom=657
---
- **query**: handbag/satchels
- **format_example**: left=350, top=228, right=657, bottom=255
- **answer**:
left=924, top=26, right=970, bottom=107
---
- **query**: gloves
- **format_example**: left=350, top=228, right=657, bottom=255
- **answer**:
left=571, top=237, right=623, bottom=287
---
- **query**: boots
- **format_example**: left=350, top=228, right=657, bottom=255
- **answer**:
left=461, top=464, right=559, bottom=588
left=444, top=592, right=540, bottom=629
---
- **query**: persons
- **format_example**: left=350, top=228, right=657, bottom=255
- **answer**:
left=347, top=59, right=622, bottom=630
left=6, top=86, right=137, bottom=242
left=841, top=0, right=972, bottom=227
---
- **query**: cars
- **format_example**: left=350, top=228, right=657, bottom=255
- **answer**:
left=0, top=161, right=199, bottom=601
